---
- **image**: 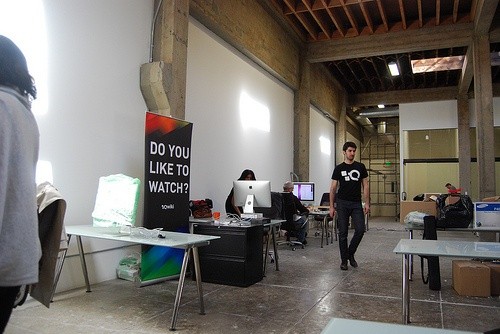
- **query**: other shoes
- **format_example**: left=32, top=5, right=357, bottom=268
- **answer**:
left=302, top=241, right=308, bottom=245
left=340, top=262, right=348, bottom=270
left=348, top=257, right=359, bottom=267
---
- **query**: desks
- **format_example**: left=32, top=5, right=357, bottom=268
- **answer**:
left=49, top=225, right=221, bottom=330
left=393, top=238, right=500, bottom=326
left=189, top=215, right=287, bottom=276
left=303, top=206, right=338, bottom=248
left=404, top=224, right=500, bottom=281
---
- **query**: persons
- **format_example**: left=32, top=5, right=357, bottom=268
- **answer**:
left=0, top=34, right=43, bottom=333
left=225, top=169, right=257, bottom=219
left=329, top=142, right=370, bottom=270
left=445, top=183, right=456, bottom=194
left=276, top=181, right=309, bottom=246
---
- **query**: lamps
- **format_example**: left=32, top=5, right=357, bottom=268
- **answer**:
left=388, top=62, right=399, bottom=77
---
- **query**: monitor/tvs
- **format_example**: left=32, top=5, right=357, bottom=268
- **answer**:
left=233, top=180, right=270, bottom=219
left=290, top=182, right=315, bottom=203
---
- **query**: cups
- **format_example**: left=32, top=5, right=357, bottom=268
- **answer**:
left=214, top=212, right=220, bottom=223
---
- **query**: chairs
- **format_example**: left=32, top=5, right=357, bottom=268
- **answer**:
left=314, top=193, right=336, bottom=237
left=255, top=191, right=305, bottom=263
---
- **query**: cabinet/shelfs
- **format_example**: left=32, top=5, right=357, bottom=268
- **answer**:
left=191, top=222, right=264, bottom=288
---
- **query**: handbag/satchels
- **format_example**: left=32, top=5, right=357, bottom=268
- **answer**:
left=436, top=194, right=474, bottom=228
left=189, top=199, right=214, bottom=219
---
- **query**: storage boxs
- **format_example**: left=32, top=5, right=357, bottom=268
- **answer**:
left=452, top=259, right=500, bottom=293
left=472, top=200, right=500, bottom=229
